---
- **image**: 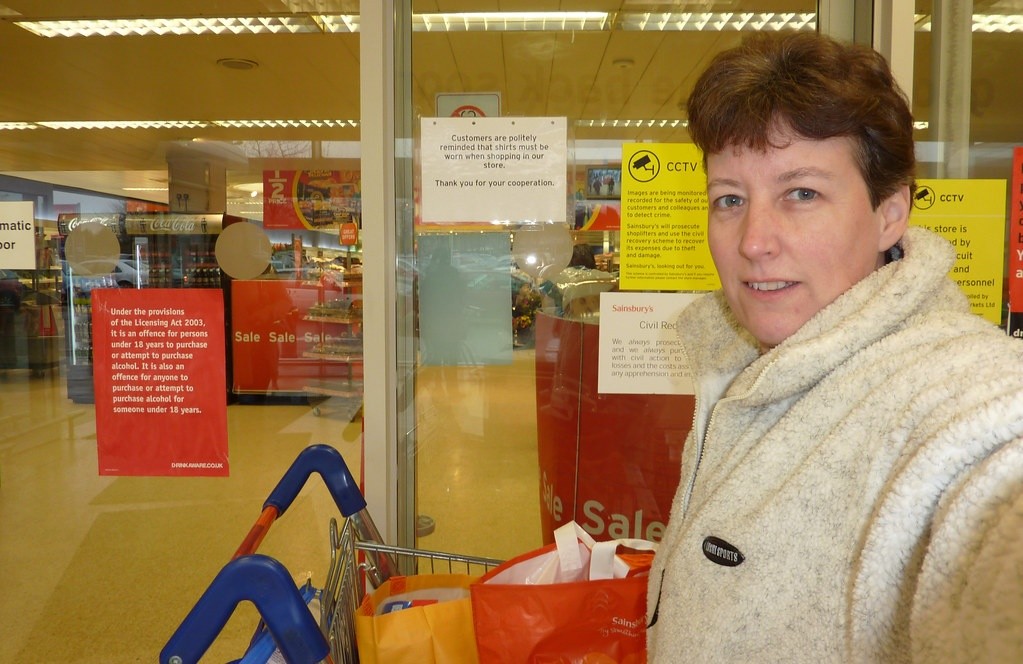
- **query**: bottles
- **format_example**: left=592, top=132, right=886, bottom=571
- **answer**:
left=187, top=238, right=216, bottom=264
left=141, top=267, right=174, bottom=288
left=326, top=296, right=352, bottom=308
left=72, top=274, right=117, bottom=298
left=184, top=264, right=223, bottom=289
left=75, top=343, right=93, bottom=362
left=132, top=236, right=173, bottom=264
left=75, top=324, right=93, bottom=342
left=74, top=303, right=92, bottom=324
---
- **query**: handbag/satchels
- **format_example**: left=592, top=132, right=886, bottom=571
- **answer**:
left=353, top=574, right=480, bottom=664
left=470, top=520, right=660, bottom=664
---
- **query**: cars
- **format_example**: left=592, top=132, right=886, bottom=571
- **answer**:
left=0, top=254, right=564, bottom=361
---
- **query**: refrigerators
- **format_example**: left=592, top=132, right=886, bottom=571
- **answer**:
left=124, top=204, right=246, bottom=405
left=59, top=211, right=131, bottom=403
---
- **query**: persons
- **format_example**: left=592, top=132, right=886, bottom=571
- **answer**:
left=648, top=29, right=1023, bottom=664
left=588, top=170, right=614, bottom=194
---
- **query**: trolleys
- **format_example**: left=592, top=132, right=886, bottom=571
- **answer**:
left=159, top=444, right=510, bottom=664
